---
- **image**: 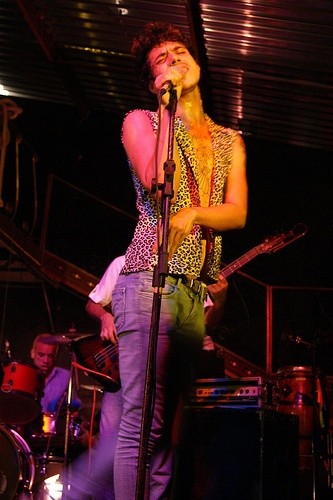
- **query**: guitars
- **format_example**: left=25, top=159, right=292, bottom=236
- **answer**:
left=68, top=220, right=308, bottom=393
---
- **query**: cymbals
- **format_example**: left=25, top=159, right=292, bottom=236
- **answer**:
left=40, top=331, right=97, bottom=343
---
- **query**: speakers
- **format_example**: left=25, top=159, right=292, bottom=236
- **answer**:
left=184, top=411, right=298, bottom=500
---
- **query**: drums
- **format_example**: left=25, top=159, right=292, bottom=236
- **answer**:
left=25, top=413, right=86, bottom=459
left=34, top=454, right=64, bottom=500
left=0, top=357, right=44, bottom=425
left=272, top=366, right=316, bottom=473
left=0, top=424, right=34, bottom=500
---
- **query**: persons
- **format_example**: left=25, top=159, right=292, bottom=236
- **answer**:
left=30, top=333, right=95, bottom=448
left=48, top=20, right=249, bottom=500
left=62, top=254, right=228, bottom=500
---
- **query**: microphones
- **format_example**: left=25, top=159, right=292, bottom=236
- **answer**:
left=281, top=333, right=332, bottom=352
left=158, top=67, right=189, bottom=95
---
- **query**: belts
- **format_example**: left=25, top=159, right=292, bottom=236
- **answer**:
left=178, top=275, right=209, bottom=300
